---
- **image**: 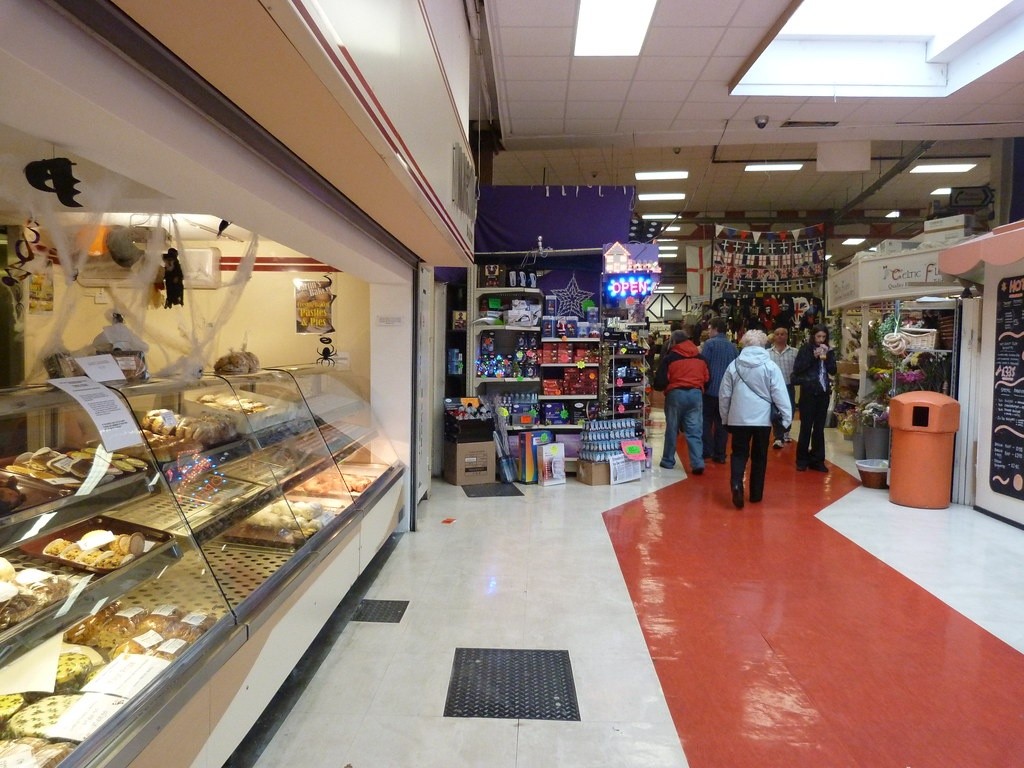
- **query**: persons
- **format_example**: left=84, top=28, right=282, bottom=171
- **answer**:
left=789, top=324, right=837, bottom=473
left=644, top=317, right=800, bottom=463
left=719, top=330, right=793, bottom=509
left=653, top=330, right=710, bottom=475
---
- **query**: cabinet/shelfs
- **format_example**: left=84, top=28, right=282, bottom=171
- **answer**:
left=0, top=356, right=408, bottom=768
left=465, top=263, right=653, bottom=473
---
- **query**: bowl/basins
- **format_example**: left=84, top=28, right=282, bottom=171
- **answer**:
left=858, top=469, right=889, bottom=489
left=854, top=459, right=889, bottom=471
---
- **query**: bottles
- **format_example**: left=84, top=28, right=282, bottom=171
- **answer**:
left=609, top=341, right=643, bottom=437
left=484, top=393, right=539, bottom=413
left=477, top=331, right=538, bottom=378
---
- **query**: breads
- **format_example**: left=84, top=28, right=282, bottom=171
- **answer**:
left=214, top=351, right=261, bottom=375
left=197, top=393, right=272, bottom=415
left=0, top=557, right=216, bottom=768
left=246, top=471, right=373, bottom=532
left=124, top=409, right=237, bottom=462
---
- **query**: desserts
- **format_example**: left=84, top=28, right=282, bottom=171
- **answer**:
left=0, top=446, right=147, bottom=569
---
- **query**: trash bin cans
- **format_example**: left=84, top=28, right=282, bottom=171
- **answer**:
left=888, top=391, right=960, bottom=509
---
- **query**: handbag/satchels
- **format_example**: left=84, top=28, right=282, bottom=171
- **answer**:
left=770, top=403, right=782, bottom=427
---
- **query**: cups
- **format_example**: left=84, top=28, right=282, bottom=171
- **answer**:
left=820, top=348, right=826, bottom=359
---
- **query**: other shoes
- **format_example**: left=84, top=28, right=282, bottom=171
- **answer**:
left=783, top=432, right=792, bottom=441
left=773, top=440, right=784, bottom=448
left=732, top=484, right=744, bottom=509
left=714, top=455, right=725, bottom=463
left=660, top=462, right=667, bottom=468
left=797, top=465, right=805, bottom=471
left=692, top=467, right=704, bottom=475
left=809, top=464, right=828, bottom=472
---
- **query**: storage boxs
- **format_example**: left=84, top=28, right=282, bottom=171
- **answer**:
left=536, top=442, right=566, bottom=486
left=577, top=459, right=616, bottom=485
left=443, top=440, right=496, bottom=485
left=517, top=430, right=550, bottom=482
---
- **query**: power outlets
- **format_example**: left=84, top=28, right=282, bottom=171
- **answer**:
left=93, top=293, right=109, bottom=305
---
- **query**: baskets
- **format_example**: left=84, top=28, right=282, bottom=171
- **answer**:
left=899, top=331, right=936, bottom=349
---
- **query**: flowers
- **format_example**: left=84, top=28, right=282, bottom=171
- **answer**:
left=869, top=314, right=925, bottom=396
left=835, top=397, right=890, bottom=435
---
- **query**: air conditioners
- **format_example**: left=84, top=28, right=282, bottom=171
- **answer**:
left=76, top=248, right=222, bottom=291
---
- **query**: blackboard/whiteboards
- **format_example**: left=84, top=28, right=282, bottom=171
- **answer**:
left=989, top=275, right=1024, bottom=501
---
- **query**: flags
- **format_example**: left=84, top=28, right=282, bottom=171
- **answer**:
left=710, top=238, right=823, bottom=331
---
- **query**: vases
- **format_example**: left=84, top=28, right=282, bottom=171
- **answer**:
left=852, top=433, right=865, bottom=460
left=863, top=426, right=889, bottom=459
